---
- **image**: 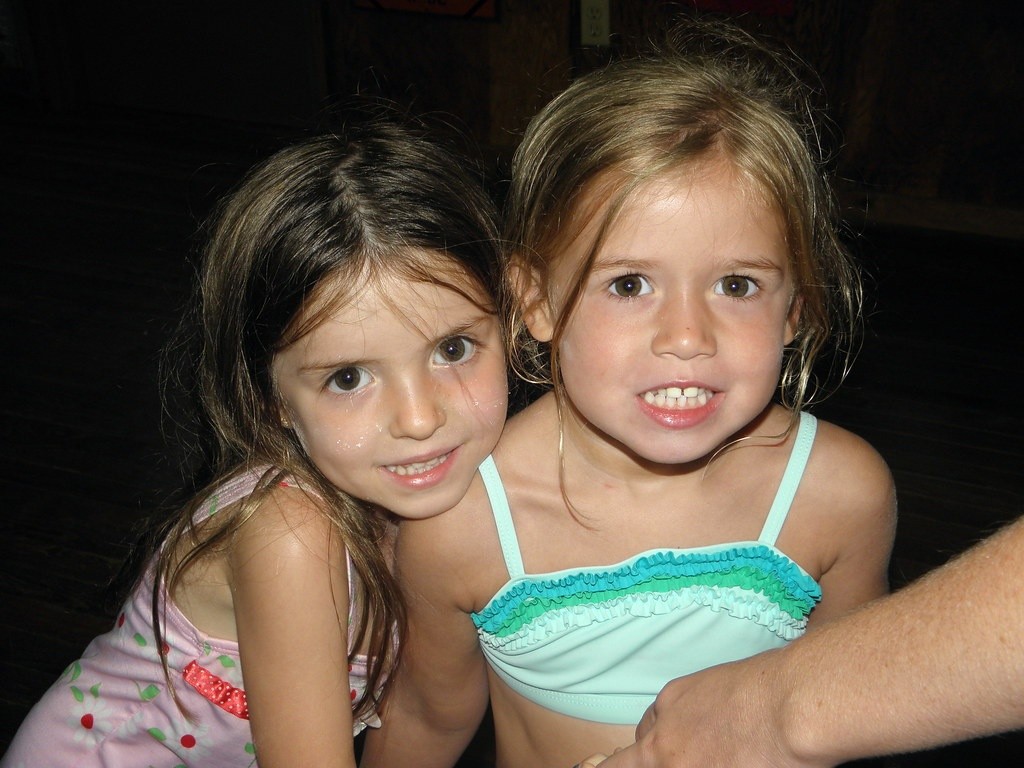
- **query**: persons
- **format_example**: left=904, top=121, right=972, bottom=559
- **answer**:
left=357, top=28, right=897, bottom=768
left=596, top=514, right=1024, bottom=768
left=1, top=117, right=509, bottom=768
